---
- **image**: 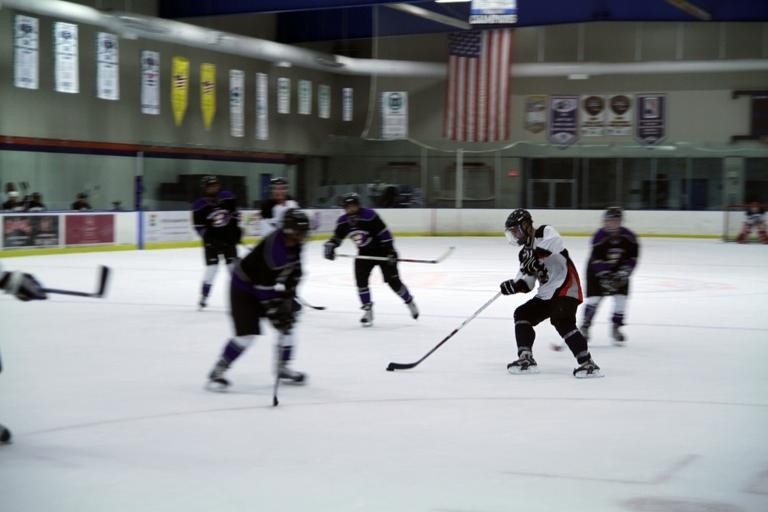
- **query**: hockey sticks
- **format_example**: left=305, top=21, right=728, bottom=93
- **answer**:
left=545, top=291, right=609, bottom=352
left=40, top=265, right=108, bottom=298
left=293, top=294, right=325, bottom=311
left=386, top=270, right=524, bottom=370
left=336, top=245, right=457, bottom=263
left=270, top=328, right=289, bottom=406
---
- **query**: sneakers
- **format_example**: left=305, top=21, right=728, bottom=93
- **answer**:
left=198, top=296, right=419, bottom=385
left=507, top=326, right=626, bottom=379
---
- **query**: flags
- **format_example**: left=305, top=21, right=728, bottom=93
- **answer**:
left=442, top=29, right=512, bottom=144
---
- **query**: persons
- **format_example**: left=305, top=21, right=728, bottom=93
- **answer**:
left=728, top=201, right=768, bottom=244
left=0, top=183, right=48, bottom=212
left=110, top=200, right=125, bottom=210
left=71, top=191, right=94, bottom=211
left=257, top=177, right=298, bottom=315
left=208, top=207, right=318, bottom=379
left=191, top=176, right=244, bottom=307
left=499, top=209, right=600, bottom=377
left=323, top=192, right=419, bottom=323
left=579, top=206, right=639, bottom=343
left=0, top=260, right=47, bottom=443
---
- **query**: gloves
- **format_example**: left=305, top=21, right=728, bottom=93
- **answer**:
left=325, top=244, right=335, bottom=261
left=1, top=272, right=47, bottom=301
left=520, top=258, right=541, bottom=276
left=501, top=280, right=518, bottom=295
left=386, top=251, right=398, bottom=267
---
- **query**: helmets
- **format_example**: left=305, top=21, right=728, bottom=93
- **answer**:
left=605, top=206, right=622, bottom=221
left=200, top=173, right=362, bottom=247
left=505, top=208, right=531, bottom=247
